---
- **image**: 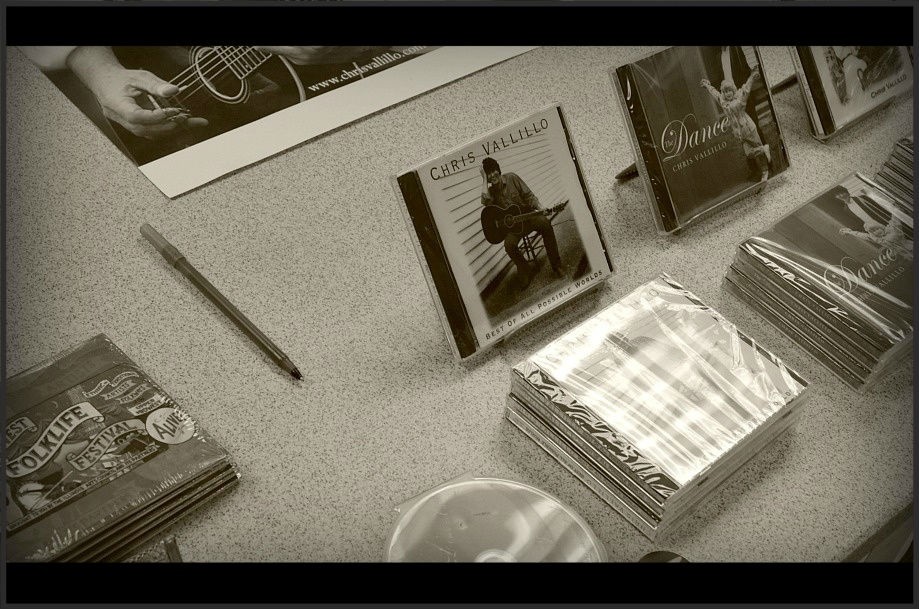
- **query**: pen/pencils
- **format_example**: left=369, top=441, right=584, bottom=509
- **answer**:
left=139, top=222, right=306, bottom=383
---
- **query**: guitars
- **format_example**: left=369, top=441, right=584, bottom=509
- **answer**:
left=91, top=43, right=307, bottom=166
left=481, top=199, right=566, bottom=245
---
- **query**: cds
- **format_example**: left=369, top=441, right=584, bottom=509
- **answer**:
left=389, top=481, right=601, bottom=562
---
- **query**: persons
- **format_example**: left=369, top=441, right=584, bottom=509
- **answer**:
left=480, top=156, right=563, bottom=289
left=699, top=47, right=772, bottom=185
left=20, top=46, right=377, bottom=142
left=839, top=208, right=912, bottom=263
left=834, top=186, right=913, bottom=232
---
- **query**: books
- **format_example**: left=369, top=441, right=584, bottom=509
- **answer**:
left=10, top=46, right=540, bottom=202
left=874, top=139, right=914, bottom=215
left=0, top=334, right=236, bottom=562
left=387, top=101, right=614, bottom=364
left=609, top=47, right=792, bottom=238
left=788, top=46, right=913, bottom=141
left=722, top=174, right=919, bottom=392
left=504, top=273, right=809, bottom=537
